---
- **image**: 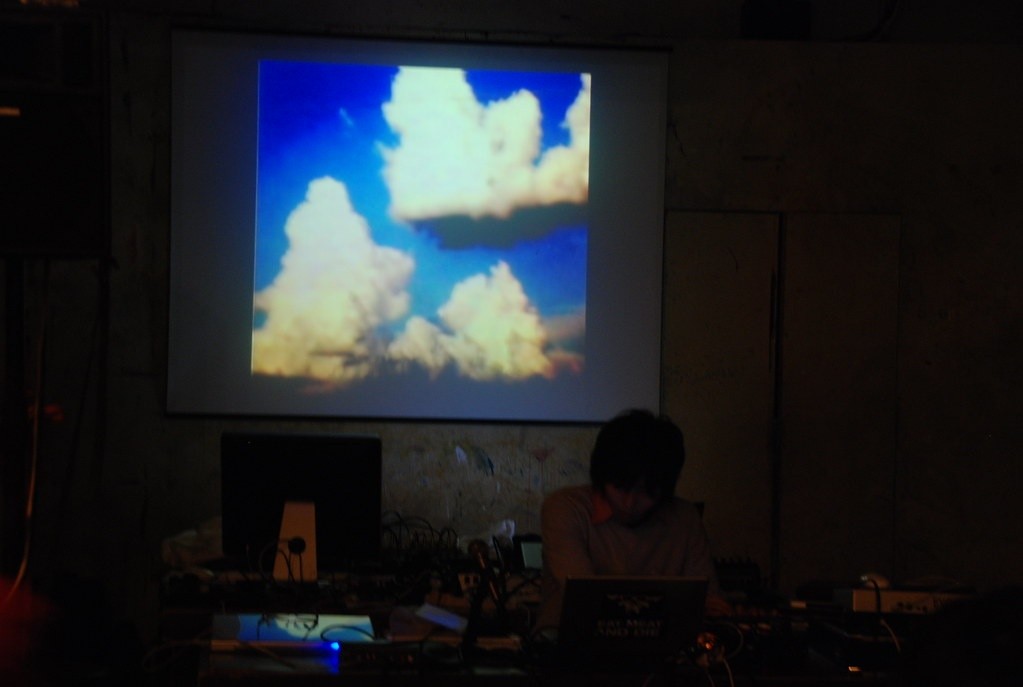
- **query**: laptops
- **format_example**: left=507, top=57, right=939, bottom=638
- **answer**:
left=541, top=574, right=709, bottom=653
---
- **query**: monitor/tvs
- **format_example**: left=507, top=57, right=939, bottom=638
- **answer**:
left=220, top=429, right=382, bottom=580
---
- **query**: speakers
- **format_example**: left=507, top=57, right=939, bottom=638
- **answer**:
left=0, top=10, right=112, bottom=260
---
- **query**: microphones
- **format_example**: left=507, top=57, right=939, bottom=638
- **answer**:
left=468, top=539, right=503, bottom=611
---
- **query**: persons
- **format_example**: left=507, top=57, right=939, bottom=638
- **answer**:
left=539, top=408, right=736, bottom=618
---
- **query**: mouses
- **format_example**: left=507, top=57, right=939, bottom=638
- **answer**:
left=858, top=572, right=890, bottom=590
